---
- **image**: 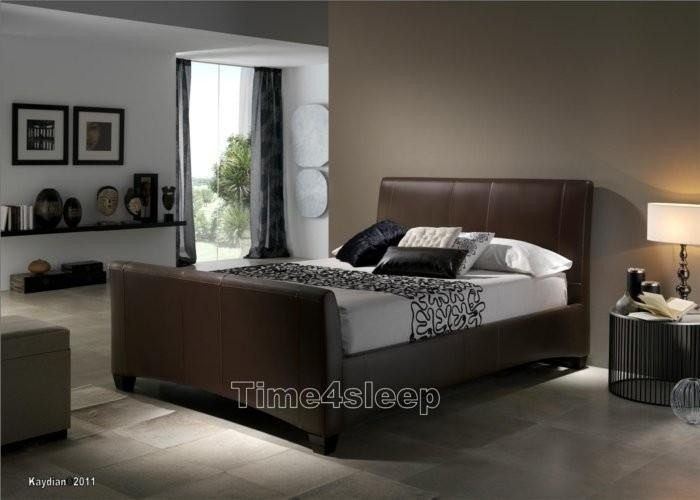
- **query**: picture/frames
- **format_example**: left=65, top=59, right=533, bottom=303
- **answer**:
left=73, top=106, right=125, bottom=165
left=12, top=102, right=69, bottom=165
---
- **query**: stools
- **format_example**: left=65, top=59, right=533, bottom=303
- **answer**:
left=1, top=316, right=71, bottom=455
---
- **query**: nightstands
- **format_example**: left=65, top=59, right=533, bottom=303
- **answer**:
left=610, top=308, right=700, bottom=407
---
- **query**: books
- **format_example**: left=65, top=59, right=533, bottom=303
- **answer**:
left=628, top=291, right=698, bottom=322
left=0, top=204, right=34, bottom=232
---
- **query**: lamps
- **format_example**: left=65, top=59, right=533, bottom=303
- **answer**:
left=646, top=203, right=700, bottom=300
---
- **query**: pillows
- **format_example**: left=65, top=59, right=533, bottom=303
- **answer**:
left=470, top=237, right=573, bottom=280
left=372, top=245, right=469, bottom=279
left=335, top=219, right=408, bottom=267
left=396, top=227, right=464, bottom=248
left=451, top=231, right=495, bottom=275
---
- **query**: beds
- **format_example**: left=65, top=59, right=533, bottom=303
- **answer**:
left=109, top=177, right=593, bottom=456
